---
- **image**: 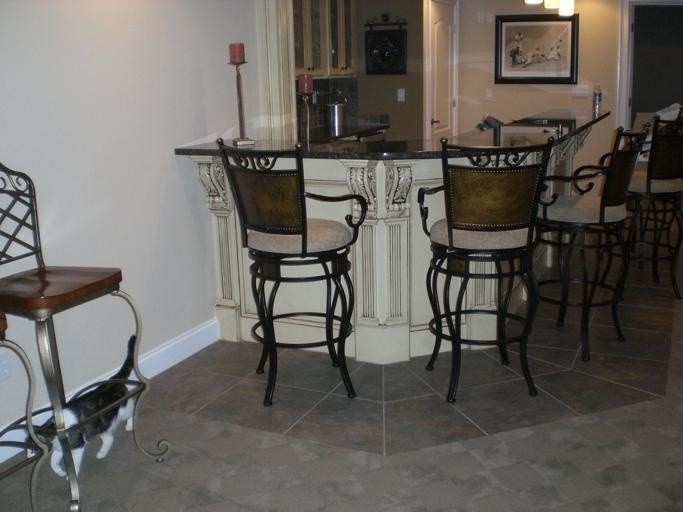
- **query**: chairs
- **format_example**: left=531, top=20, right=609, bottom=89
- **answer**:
left=533, top=126, right=652, bottom=366
left=0, top=162, right=171, bottom=511
left=622, top=111, right=681, bottom=294
left=413, top=133, right=554, bottom=405
left=215, top=137, right=369, bottom=407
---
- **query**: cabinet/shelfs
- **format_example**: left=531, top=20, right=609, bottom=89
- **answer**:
left=328, top=0, right=356, bottom=79
left=292, top=0, right=327, bottom=79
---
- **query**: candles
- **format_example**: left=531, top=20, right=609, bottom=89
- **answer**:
left=298, top=74, right=311, bottom=93
left=227, top=41, right=244, bottom=62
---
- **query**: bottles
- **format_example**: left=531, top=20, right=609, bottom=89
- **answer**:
left=590, top=82, right=603, bottom=113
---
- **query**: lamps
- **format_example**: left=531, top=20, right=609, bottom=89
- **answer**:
left=524, top=0, right=575, bottom=19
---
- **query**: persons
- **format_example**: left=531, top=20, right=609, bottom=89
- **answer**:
left=510, top=31, right=563, bottom=68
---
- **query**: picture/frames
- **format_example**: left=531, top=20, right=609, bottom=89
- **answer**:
left=493, top=13, right=580, bottom=87
left=364, top=30, right=407, bottom=77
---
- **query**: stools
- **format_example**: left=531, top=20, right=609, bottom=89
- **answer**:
left=623, top=158, right=677, bottom=271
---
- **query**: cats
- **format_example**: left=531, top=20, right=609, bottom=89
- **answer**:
left=25, top=334, right=138, bottom=480
